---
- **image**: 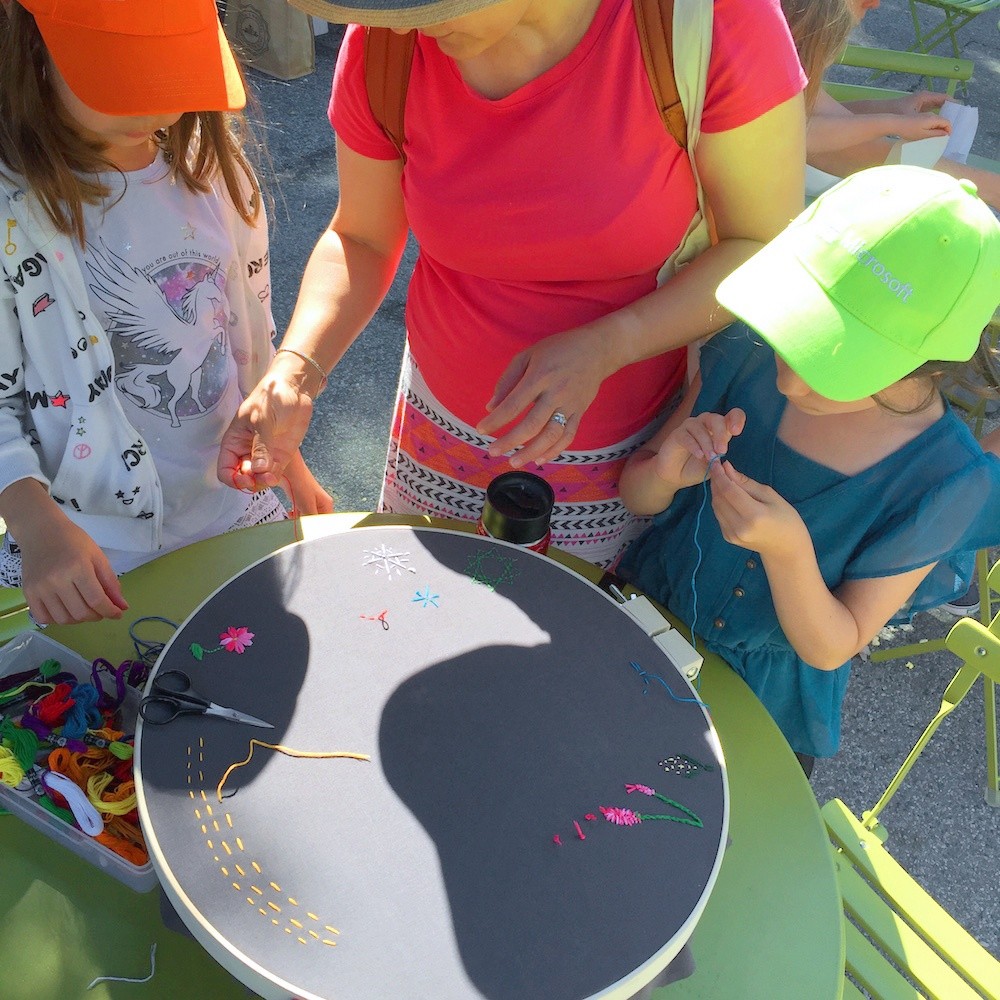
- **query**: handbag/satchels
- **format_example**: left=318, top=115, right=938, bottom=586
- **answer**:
left=224, top=0, right=316, bottom=82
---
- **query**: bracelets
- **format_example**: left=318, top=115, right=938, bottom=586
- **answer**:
left=273, top=348, right=327, bottom=381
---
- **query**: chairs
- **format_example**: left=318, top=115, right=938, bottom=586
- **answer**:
left=818, top=0, right=1000, bottom=1000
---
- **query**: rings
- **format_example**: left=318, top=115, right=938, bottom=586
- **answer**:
left=550, top=412, right=567, bottom=427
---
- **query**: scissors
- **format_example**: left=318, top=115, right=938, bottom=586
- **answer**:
left=137, top=669, right=275, bottom=728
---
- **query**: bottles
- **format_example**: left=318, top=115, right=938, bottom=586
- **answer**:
left=475, top=470, right=555, bottom=556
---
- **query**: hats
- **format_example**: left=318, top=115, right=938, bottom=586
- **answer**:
left=18, top=0, right=247, bottom=117
left=714, top=164, right=1000, bottom=404
left=286, top=0, right=508, bottom=28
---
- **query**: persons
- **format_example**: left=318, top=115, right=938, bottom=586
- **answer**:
left=1, top=0, right=334, bottom=626
left=781, top=0, right=1000, bottom=216
left=197, top=0, right=809, bottom=588
left=615, top=163, right=1000, bottom=785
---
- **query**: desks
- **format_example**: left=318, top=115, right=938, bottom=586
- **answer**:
left=0, top=513, right=848, bottom=1000
